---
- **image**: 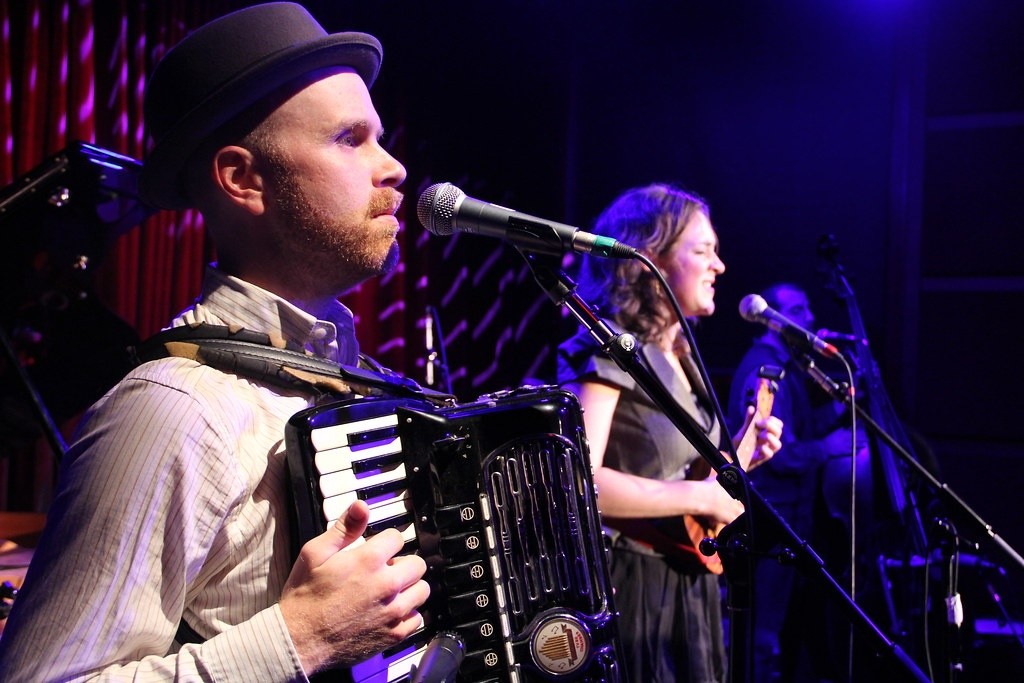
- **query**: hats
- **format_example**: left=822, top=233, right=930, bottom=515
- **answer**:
left=144, top=2, right=382, bottom=178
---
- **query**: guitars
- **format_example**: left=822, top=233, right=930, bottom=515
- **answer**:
left=665, top=360, right=785, bottom=574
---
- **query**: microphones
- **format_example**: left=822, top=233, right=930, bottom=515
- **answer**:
left=417, top=181, right=637, bottom=260
left=738, top=293, right=840, bottom=359
left=817, top=328, right=866, bottom=346
left=412, top=631, right=465, bottom=683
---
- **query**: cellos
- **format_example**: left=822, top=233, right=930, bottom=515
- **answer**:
left=819, top=230, right=958, bottom=683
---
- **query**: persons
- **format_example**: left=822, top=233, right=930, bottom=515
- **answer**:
left=731, top=282, right=902, bottom=683
left=0, top=1, right=431, bottom=683
left=555, top=187, right=784, bottom=683
